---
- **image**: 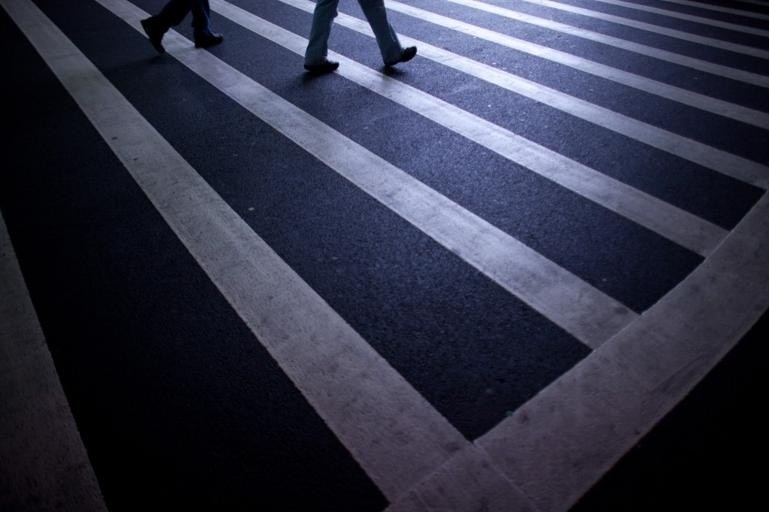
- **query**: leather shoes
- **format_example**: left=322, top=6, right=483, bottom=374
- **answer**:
left=385, top=46, right=416, bottom=66
left=140, top=17, right=165, bottom=52
left=305, top=60, right=338, bottom=72
left=195, top=34, right=223, bottom=48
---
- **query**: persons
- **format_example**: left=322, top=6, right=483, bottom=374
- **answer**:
left=141, top=0, right=223, bottom=53
left=303, top=0, right=416, bottom=72
